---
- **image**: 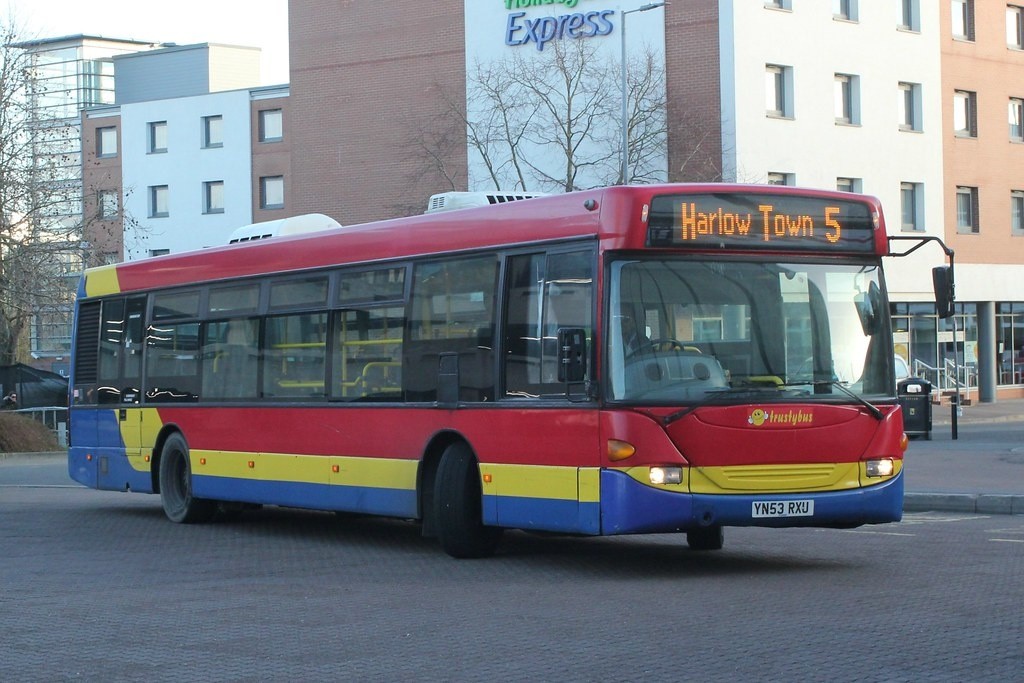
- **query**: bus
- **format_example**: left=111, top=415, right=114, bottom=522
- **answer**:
left=64, top=180, right=952, bottom=558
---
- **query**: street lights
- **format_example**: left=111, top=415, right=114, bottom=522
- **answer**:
left=621, top=2, right=671, bottom=184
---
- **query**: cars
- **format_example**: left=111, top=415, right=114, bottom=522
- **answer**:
left=793, top=353, right=915, bottom=395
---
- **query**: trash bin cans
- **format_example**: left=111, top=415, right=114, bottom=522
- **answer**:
left=897, top=375, right=933, bottom=439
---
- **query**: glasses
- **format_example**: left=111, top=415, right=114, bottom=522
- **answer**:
left=620, top=312, right=633, bottom=325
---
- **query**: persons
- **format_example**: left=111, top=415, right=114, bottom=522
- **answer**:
left=0, top=391, right=21, bottom=410
left=621, top=302, right=652, bottom=358
